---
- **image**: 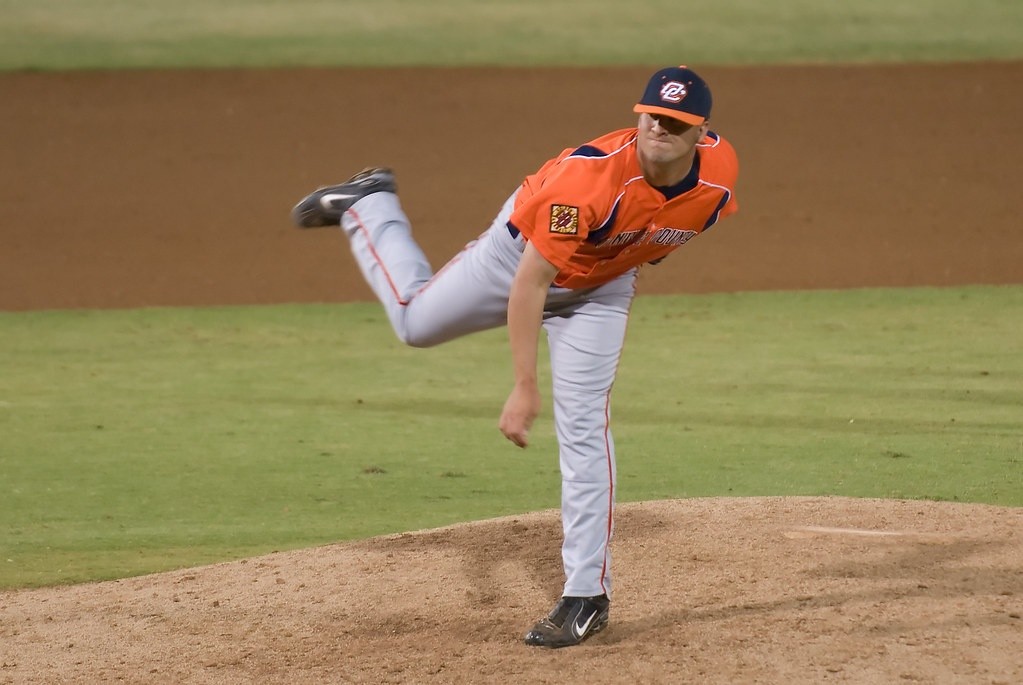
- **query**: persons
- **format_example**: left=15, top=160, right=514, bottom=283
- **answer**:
left=290, top=63, right=741, bottom=647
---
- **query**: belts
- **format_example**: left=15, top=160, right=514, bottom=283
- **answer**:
left=506, top=222, right=520, bottom=239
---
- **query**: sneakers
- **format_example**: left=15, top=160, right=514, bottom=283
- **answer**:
left=290, top=167, right=398, bottom=228
left=524, top=594, right=610, bottom=648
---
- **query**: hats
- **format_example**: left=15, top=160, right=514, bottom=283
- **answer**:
left=632, top=65, right=712, bottom=126
